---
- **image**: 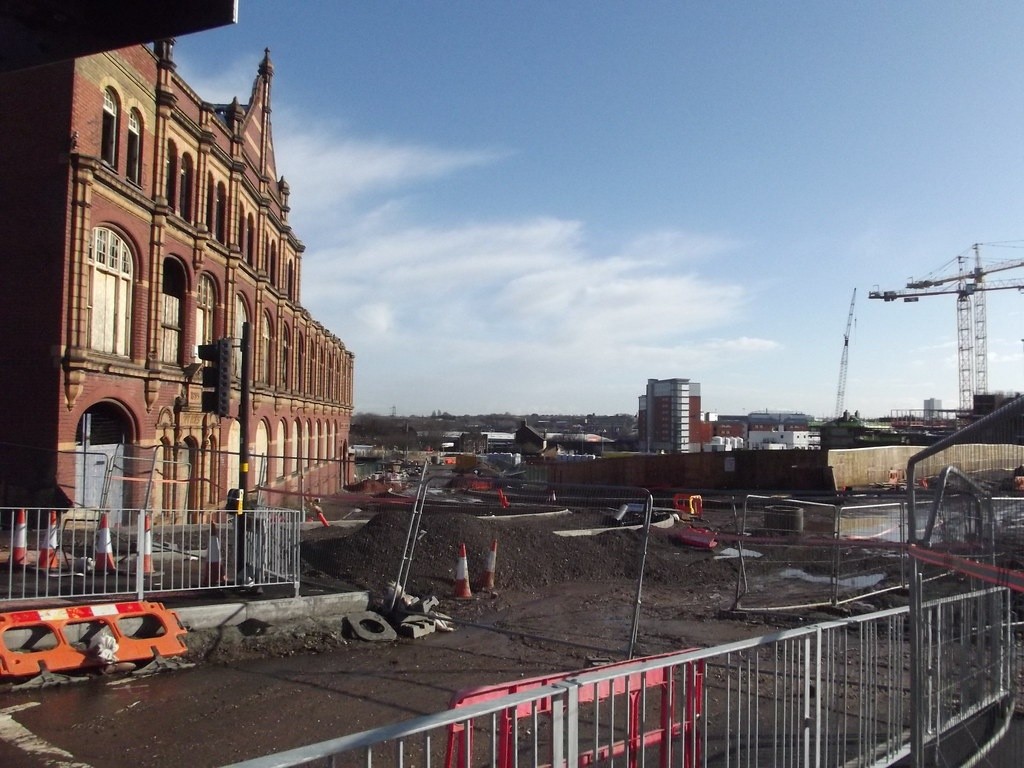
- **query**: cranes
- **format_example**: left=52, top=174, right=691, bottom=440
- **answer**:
left=868, top=255, right=1024, bottom=414
left=835, top=288, right=857, bottom=419
left=906, top=241, right=1024, bottom=395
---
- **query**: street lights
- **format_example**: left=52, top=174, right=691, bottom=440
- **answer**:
left=602, top=430, right=607, bottom=454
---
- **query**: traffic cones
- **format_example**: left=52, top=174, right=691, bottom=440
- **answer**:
left=446, top=543, right=475, bottom=600
left=143, top=514, right=159, bottom=570
left=203, top=521, right=226, bottom=586
left=6, top=508, right=31, bottom=566
left=95, top=514, right=116, bottom=572
left=38, top=511, right=58, bottom=569
left=473, top=539, right=497, bottom=590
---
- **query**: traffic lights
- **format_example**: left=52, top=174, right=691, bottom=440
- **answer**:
left=198, top=340, right=230, bottom=417
